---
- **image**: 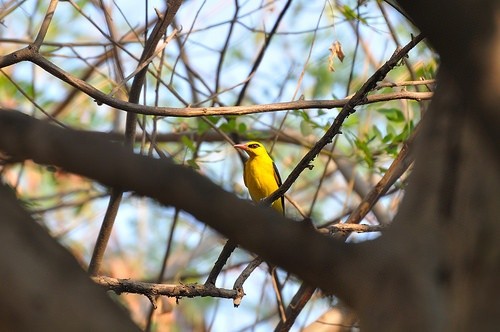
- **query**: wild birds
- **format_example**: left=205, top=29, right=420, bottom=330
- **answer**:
left=234, top=140, right=287, bottom=218
